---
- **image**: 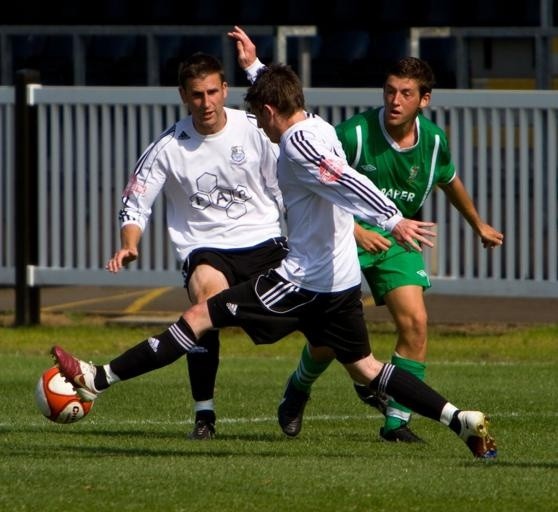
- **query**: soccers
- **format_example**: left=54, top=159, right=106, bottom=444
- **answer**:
left=36, top=364, right=96, bottom=424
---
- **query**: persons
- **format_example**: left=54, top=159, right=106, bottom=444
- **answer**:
left=276, top=55, right=505, bottom=445
left=104, top=51, right=387, bottom=441
left=50, top=25, right=497, bottom=460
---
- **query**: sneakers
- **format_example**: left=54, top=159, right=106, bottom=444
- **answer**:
left=50, top=345, right=106, bottom=402
left=378, top=425, right=428, bottom=445
left=456, top=409, right=498, bottom=461
left=277, top=375, right=310, bottom=438
left=189, top=418, right=216, bottom=442
